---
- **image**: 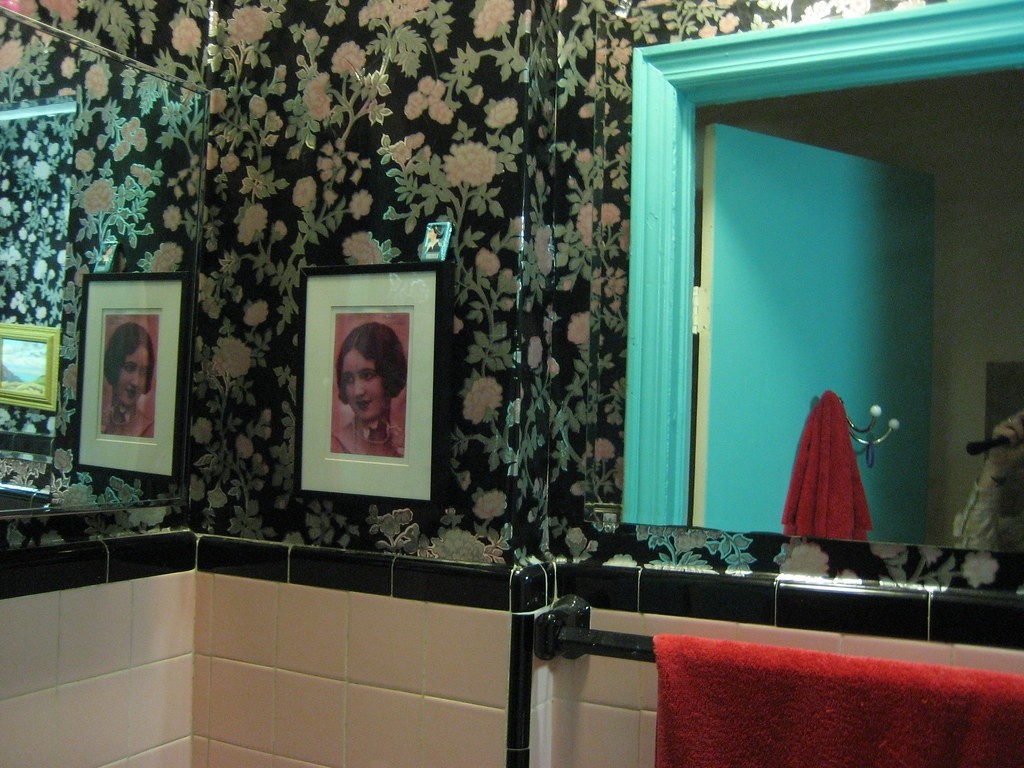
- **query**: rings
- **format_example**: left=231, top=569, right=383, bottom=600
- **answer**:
left=1008, top=416, right=1012, bottom=423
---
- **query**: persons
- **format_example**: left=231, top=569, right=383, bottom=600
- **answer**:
left=952, top=409, right=1024, bottom=553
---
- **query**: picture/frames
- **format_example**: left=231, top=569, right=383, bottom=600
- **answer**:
left=0, top=320, right=62, bottom=411
left=72, top=270, right=193, bottom=482
left=298, top=263, right=444, bottom=505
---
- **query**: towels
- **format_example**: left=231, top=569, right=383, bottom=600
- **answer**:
left=652, top=632, right=1022, bottom=768
left=782, top=388, right=869, bottom=542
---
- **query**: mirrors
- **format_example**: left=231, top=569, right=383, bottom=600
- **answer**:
left=0, top=5, right=211, bottom=521
left=586, top=0, right=1024, bottom=554
